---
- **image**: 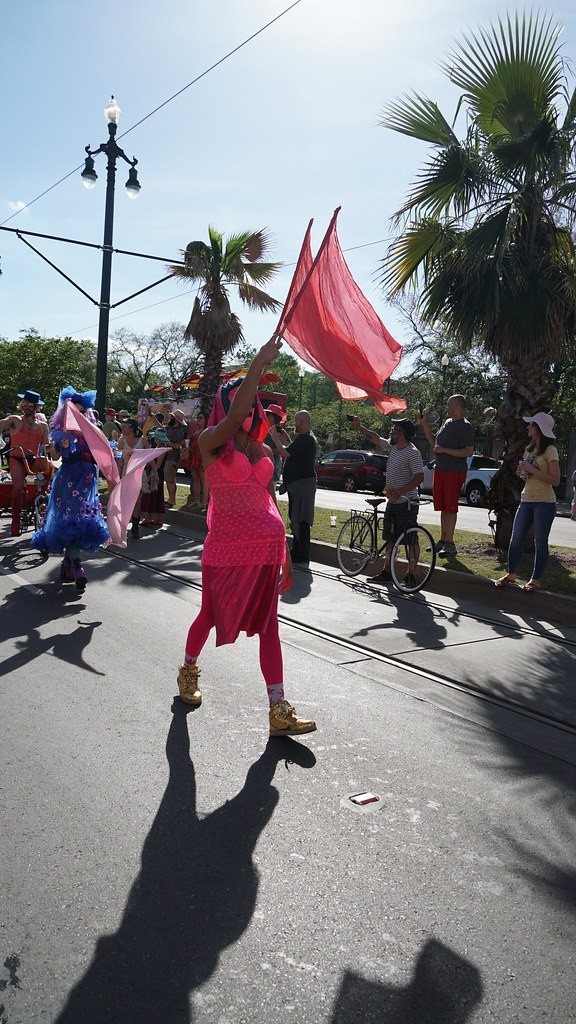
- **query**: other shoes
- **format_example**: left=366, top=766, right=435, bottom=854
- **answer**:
left=288, top=549, right=309, bottom=564
left=139, top=500, right=208, bottom=525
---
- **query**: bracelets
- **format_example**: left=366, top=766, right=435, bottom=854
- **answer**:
left=531, top=469, right=534, bottom=475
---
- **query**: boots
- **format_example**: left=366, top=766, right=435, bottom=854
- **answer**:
left=72, top=558, right=87, bottom=589
left=131, top=514, right=141, bottom=539
left=39, top=471, right=52, bottom=496
left=60, top=550, right=74, bottom=578
left=12, top=504, right=21, bottom=535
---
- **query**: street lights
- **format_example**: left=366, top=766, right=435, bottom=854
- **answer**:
left=79, top=93, right=141, bottom=424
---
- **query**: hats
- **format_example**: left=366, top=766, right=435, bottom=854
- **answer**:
left=392, top=418, right=415, bottom=436
left=103, top=409, right=115, bottom=415
left=17, top=390, right=45, bottom=406
left=522, top=412, right=556, bottom=439
left=263, top=404, right=288, bottom=423
left=148, top=408, right=187, bottom=443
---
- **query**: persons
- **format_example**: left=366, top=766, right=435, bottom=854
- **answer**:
left=571, top=468, right=576, bottom=521
left=417, top=394, right=474, bottom=556
left=30, top=385, right=109, bottom=590
left=496, top=413, right=560, bottom=591
left=0, top=387, right=292, bottom=539
left=352, top=416, right=424, bottom=587
left=269, top=409, right=318, bottom=567
left=176, top=330, right=316, bottom=737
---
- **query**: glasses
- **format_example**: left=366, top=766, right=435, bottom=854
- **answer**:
left=122, top=415, right=129, bottom=418
left=197, top=416, right=204, bottom=419
left=79, top=410, right=85, bottom=414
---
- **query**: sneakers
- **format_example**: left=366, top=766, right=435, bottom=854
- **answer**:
left=426, top=539, right=457, bottom=556
left=177, top=664, right=202, bottom=704
left=400, top=572, right=417, bottom=587
left=367, top=570, right=393, bottom=583
left=269, top=699, right=316, bottom=736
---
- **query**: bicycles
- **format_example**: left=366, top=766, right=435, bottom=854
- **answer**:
left=336, top=493, right=436, bottom=594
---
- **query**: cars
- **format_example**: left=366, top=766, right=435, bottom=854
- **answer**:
left=416, top=454, right=504, bottom=507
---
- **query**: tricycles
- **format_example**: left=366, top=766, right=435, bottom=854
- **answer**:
left=0, top=444, right=56, bottom=555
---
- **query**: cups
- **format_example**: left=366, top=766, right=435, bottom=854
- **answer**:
left=519, top=460, right=528, bottom=479
left=330, top=514, right=337, bottom=526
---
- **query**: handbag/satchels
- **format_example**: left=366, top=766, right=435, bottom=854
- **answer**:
left=10, top=454, right=36, bottom=465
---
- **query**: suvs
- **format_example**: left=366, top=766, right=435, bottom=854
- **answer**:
left=313, top=449, right=389, bottom=494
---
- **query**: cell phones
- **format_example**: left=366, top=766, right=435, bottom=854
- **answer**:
left=419, top=403, right=423, bottom=419
left=346, top=414, right=354, bottom=422
left=275, top=426, right=283, bottom=433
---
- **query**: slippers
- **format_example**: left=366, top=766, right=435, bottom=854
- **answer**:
left=495, top=577, right=539, bottom=591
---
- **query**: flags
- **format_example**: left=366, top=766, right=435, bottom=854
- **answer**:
left=104, top=446, right=170, bottom=547
left=62, top=396, right=120, bottom=483
left=287, top=216, right=409, bottom=414
left=278, top=230, right=372, bottom=401
